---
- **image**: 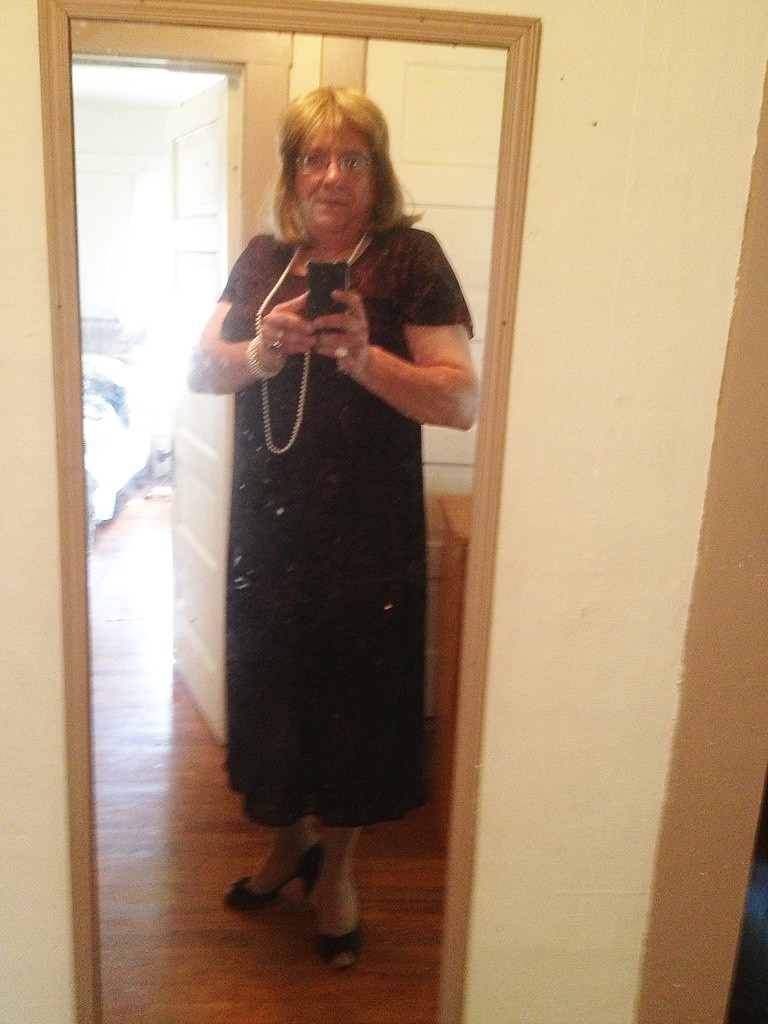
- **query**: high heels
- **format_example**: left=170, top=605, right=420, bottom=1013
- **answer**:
left=222, top=837, right=323, bottom=910
left=315, top=910, right=361, bottom=971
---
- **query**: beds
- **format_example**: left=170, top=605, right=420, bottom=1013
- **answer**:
left=80, top=338, right=162, bottom=531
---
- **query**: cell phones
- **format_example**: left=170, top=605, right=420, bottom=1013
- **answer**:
left=304, top=259, right=351, bottom=335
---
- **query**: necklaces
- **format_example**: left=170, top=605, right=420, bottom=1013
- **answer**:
left=253, top=229, right=369, bottom=454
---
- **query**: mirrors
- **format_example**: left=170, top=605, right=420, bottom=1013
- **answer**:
left=34, top=0, right=546, bottom=1023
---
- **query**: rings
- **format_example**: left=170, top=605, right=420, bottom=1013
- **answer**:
left=275, top=329, right=285, bottom=340
left=335, top=346, right=349, bottom=358
left=270, top=341, right=283, bottom=351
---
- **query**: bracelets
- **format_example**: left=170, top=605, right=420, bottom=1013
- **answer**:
left=351, top=345, right=372, bottom=373
left=245, top=336, right=279, bottom=379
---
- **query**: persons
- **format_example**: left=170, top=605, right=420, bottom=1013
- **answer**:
left=185, top=84, right=478, bottom=965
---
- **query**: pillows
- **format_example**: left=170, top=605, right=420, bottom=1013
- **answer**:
left=81, top=317, right=147, bottom=361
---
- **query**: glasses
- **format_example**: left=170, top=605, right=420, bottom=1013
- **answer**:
left=293, top=154, right=371, bottom=177
